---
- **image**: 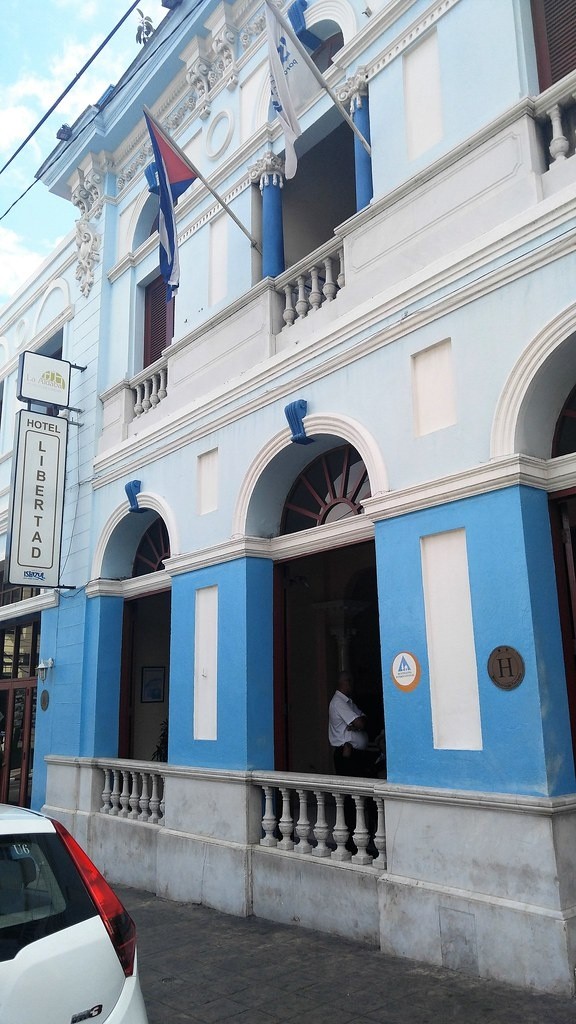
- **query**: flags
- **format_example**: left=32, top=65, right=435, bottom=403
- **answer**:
left=265, top=0, right=328, bottom=179
left=143, top=105, right=197, bottom=303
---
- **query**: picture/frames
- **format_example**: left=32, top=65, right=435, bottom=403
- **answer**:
left=141, top=666, right=166, bottom=703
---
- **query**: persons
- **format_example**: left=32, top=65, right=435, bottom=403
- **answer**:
left=329, top=671, right=386, bottom=848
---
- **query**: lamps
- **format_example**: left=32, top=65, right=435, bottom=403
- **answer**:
left=35, top=658, right=54, bottom=685
left=56, top=124, right=72, bottom=141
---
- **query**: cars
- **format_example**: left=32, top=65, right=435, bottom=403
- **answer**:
left=0, top=800, right=149, bottom=1024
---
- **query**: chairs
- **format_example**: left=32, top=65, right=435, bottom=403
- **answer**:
left=0, top=857, right=52, bottom=915
